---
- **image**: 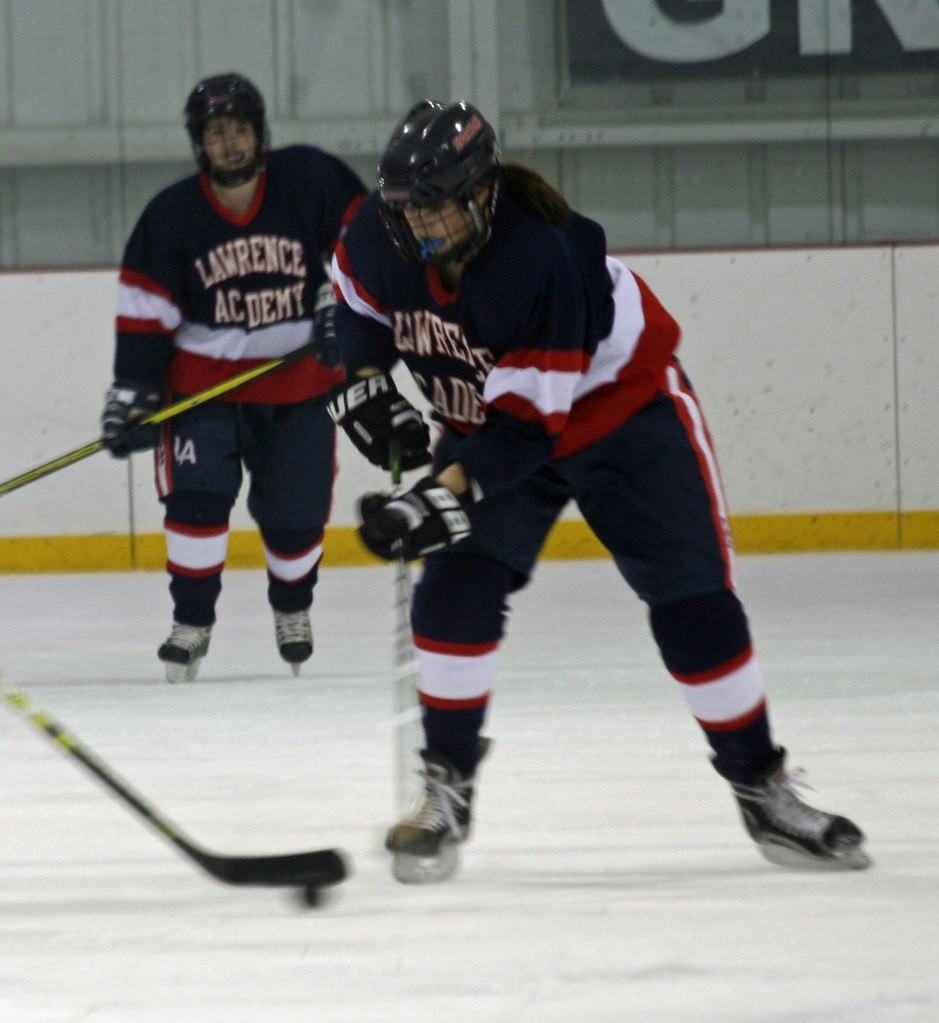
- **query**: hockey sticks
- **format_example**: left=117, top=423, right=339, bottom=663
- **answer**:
left=0, top=342, right=317, bottom=497
left=1, top=672, right=347, bottom=888
left=388, top=434, right=420, bottom=816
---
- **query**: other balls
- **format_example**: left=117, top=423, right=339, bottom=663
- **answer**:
left=304, top=875, right=321, bottom=908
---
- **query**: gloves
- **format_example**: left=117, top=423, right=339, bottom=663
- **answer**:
left=101, top=384, right=170, bottom=461
left=321, top=370, right=436, bottom=471
left=353, top=476, right=472, bottom=558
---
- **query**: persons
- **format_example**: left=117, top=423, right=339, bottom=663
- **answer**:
left=309, top=99, right=873, bottom=882
left=100, top=72, right=367, bottom=685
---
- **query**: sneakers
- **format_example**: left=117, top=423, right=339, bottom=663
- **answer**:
left=711, top=745, right=873, bottom=874
left=156, top=620, right=213, bottom=685
left=384, top=736, right=493, bottom=884
left=274, top=610, right=316, bottom=676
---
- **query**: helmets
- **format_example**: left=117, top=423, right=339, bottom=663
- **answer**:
left=379, top=99, right=502, bottom=268
left=185, top=71, right=269, bottom=186
